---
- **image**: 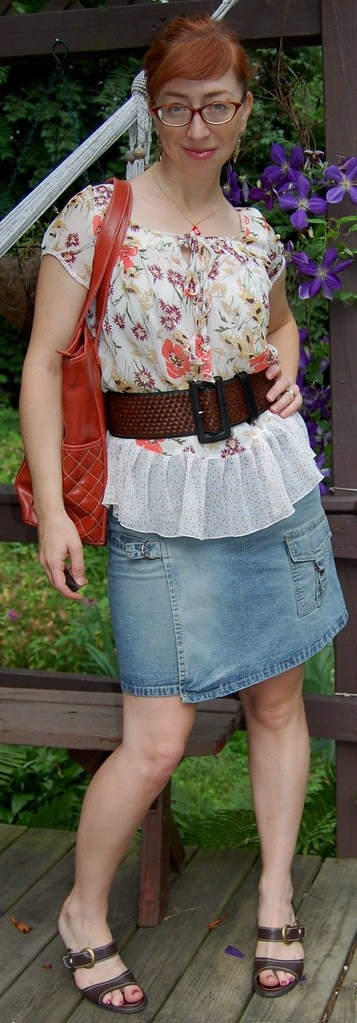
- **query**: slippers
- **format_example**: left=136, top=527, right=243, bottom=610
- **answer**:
left=56, top=914, right=148, bottom=1013
left=251, top=900, right=305, bottom=997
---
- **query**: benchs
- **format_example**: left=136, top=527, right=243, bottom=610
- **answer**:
left=0, top=687, right=243, bottom=928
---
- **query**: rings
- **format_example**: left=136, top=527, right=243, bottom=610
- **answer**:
left=287, top=389, right=295, bottom=398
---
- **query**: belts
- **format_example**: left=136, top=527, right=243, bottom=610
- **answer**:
left=104, top=368, right=283, bottom=440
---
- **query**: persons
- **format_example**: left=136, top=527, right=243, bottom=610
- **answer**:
left=20, top=17, right=350, bottom=1016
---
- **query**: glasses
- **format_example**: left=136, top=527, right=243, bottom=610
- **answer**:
left=149, top=83, right=246, bottom=127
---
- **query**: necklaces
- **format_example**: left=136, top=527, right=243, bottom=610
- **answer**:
left=148, top=165, right=224, bottom=236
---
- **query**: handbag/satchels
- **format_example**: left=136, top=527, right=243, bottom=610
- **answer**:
left=14, top=177, right=134, bottom=547
